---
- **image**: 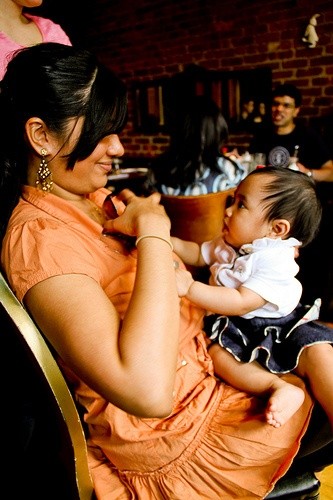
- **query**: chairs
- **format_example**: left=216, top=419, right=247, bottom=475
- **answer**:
left=158, top=187, right=237, bottom=247
left=0, top=270, right=96, bottom=500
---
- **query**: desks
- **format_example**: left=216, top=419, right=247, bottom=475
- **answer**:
left=108, top=166, right=149, bottom=182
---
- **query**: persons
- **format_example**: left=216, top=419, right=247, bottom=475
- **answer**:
left=0, top=0, right=74, bottom=89
left=240, top=98, right=255, bottom=120
left=251, top=83, right=333, bottom=182
left=0, top=41, right=333, bottom=500
left=143, top=97, right=243, bottom=202
left=293, top=159, right=333, bottom=181
left=171, top=166, right=333, bottom=428
left=252, top=102, right=267, bottom=124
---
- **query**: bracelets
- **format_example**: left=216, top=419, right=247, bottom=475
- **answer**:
left=136, top=234, right=173, bottom=250
left=307, top=169, right=313, bottom=178
